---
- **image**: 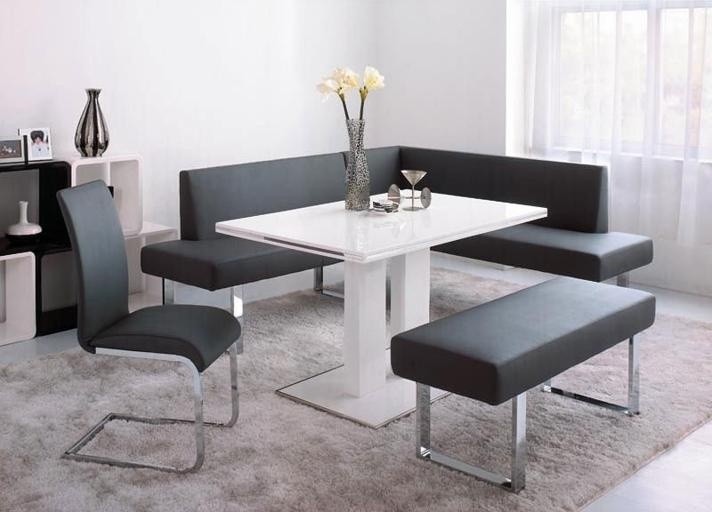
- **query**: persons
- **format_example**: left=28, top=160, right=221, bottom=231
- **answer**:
left=30, top=130, right=49, bottom=158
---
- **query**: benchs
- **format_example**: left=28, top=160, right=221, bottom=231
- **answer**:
left=391, top=277, right=658, bottom=495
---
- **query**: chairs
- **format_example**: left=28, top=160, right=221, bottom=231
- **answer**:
left=56, top=180, right=246, bottom=477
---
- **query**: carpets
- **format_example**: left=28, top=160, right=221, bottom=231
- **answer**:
left=1, top=268, right=712, bottom=512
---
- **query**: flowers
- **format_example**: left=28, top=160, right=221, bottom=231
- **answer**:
left=316, top=62, right=387, bottom=148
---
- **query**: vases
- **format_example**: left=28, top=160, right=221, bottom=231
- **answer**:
left=345, top=119, right=370, bottom=212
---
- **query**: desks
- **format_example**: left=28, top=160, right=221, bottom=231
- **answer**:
left=211, top=188, right=549, bottom=432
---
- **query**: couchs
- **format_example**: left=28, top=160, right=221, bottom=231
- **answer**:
left=140, top=145, right=655, bottom=319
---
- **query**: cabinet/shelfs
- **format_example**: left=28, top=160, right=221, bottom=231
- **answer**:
left=1, top=154, right=177, bottom=344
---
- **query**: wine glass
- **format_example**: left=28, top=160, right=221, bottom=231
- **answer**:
left=388, top=170, right=432, bottom=212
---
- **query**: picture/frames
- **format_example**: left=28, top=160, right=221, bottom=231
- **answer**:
left=1, top=127, right=53, bottom=163
